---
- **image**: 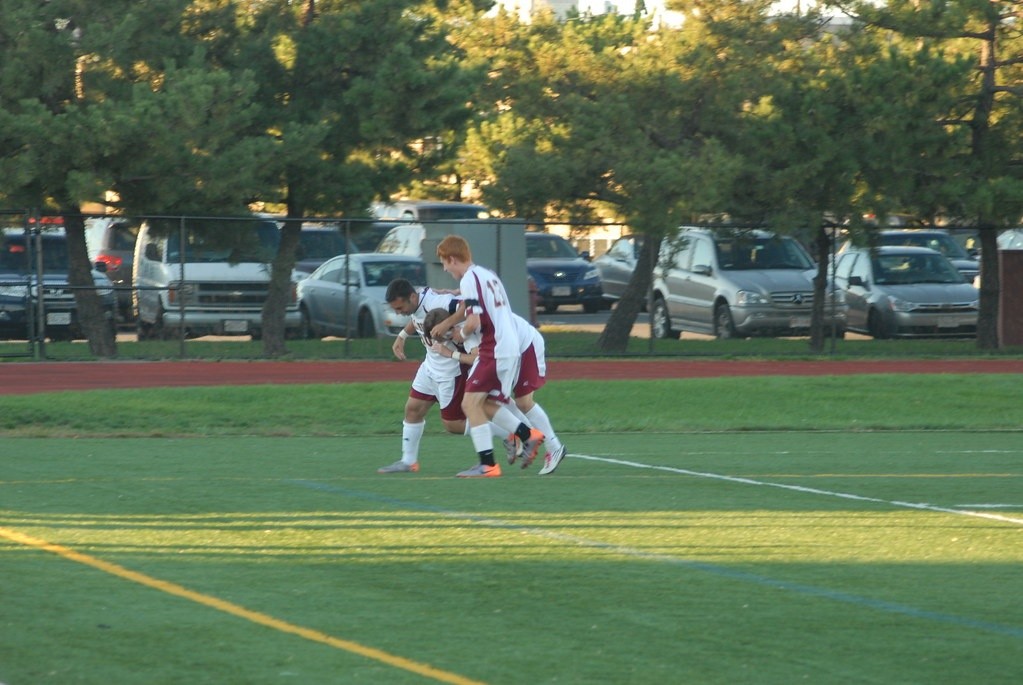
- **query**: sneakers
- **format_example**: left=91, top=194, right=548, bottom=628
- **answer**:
left=516, top=440, right=524, bottom=456
left=503, top=433, right=519, bottom=465
left=378, top=460, right=419, bottom=473
left=537, top=444, right=567, bottom=475
left=520, top=430, right=546, bottom=468
left=455, top=462, right=501, bottom=478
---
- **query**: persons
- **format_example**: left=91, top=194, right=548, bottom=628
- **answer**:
left=424, top=308, right=567, bottom=474
left=378, top=279, right=520, bottom=474
left=434, top=234, right=546, bottom=477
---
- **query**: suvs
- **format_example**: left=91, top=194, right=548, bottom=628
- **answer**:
left=645, top=224, right=851, bottom=339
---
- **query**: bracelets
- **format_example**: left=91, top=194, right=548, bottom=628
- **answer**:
left=451, top=351, right=462, bottom=360
left=460, top=329, right=470, bottom=340
left=398, top=329, right=409, bottom=340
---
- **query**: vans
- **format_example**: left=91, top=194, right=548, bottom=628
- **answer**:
left=373, top=200, right=493, bottom=220
left=96, top=214, right=303, bottom=344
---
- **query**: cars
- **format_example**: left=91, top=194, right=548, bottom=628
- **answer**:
left=524, top=232, right=646, bottom=313
left=0, top=227, right=119, bottom=342
left=275, top=225, right=427, bottom=339
left=829, top=229, right=982, bottom=340
